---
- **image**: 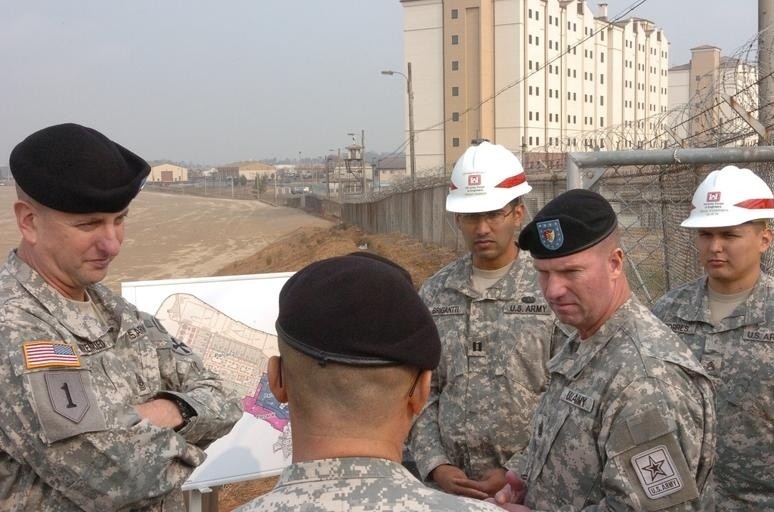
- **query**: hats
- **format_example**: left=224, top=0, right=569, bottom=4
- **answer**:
left=518, top=188, right=618, bottom=259
left=275, top=251, right=442, bottom=371
left=9, top=123, right=152, bottom=213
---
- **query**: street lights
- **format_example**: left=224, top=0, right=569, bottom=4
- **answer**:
left=225, top=145, right=342, bottom=203
left=380, top=61, right=414, bottom=181
left=345, top=129, right=371, bottom=195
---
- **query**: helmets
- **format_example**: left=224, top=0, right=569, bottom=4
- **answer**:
left=679, top=165, right=770, bottom=229
left=445, top=141, right=533, bottom=214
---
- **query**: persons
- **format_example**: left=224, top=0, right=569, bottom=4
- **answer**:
left=225, top=253, right=499, bottom=512
left=490, top=186, right=715, bottom=512
left=0, top=120, right=242, bottom=512
left=641, top=165, right=772, bottom=512
left=408, top=144, right=564, bottom=501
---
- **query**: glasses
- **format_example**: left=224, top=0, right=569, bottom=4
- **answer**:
left=455, top=202, right=521, bottom=225
